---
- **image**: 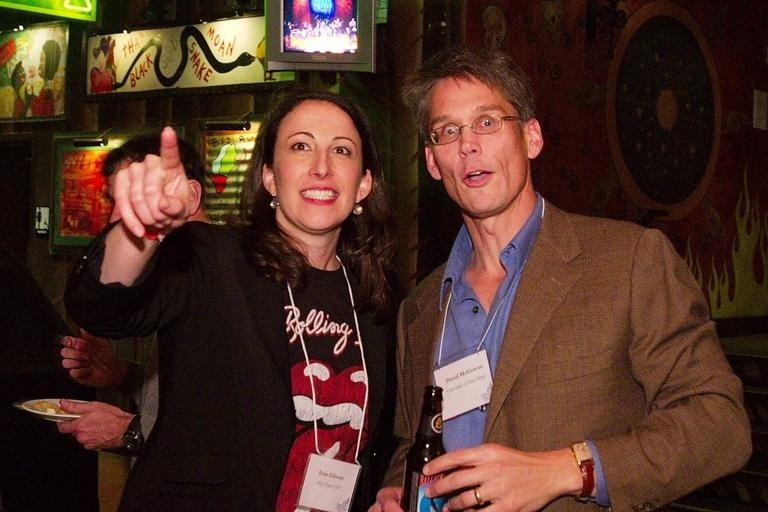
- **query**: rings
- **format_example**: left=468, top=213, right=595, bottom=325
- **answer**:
left=474, top=487, right=483, bottom=506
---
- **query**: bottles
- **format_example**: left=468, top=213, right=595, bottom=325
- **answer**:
left=401, top=385, right=452, bottom=512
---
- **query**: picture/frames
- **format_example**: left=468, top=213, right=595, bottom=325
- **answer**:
left=77, top=8, right=306, bottom=103
left=47, top=124, right=189, bottom=256
left=0, top=18, right=73, bottom=124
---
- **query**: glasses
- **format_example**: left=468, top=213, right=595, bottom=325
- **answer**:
left=429, top=114, right=522, bottom=145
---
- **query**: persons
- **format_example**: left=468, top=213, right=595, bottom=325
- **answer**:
left=366, top=43, right=754, bottom=512
left=63, top=91, right=402, bottom=512
left=56, top=133, right=211, bottom=454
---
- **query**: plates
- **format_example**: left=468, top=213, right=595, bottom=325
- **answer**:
left=14, top=398, right=95, bottom=422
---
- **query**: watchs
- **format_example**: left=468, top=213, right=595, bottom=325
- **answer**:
left=122, top=415, right=144, bottom=453
left=569, top=440, right=594, bottom=503
